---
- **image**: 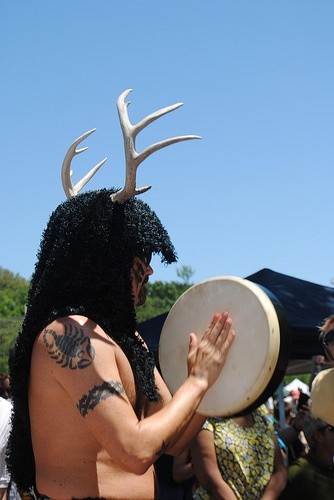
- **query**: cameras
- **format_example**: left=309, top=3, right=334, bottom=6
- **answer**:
left=297, top=392, right=310, bottom=410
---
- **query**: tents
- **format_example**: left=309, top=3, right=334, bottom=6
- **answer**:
left=136, top=268, right=334, bottom=428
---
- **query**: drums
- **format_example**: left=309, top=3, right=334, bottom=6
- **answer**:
left=158, top=275, right=286, bottom=422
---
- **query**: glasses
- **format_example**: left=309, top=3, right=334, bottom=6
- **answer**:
left=323, top=329, right=334, bottom=346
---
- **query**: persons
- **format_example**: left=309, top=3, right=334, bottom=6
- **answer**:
left=154, top=315, right=334, bottom=500
left=4, top=187, right=237, bottom=500
left=0, top=374, right=37, bottom=500
